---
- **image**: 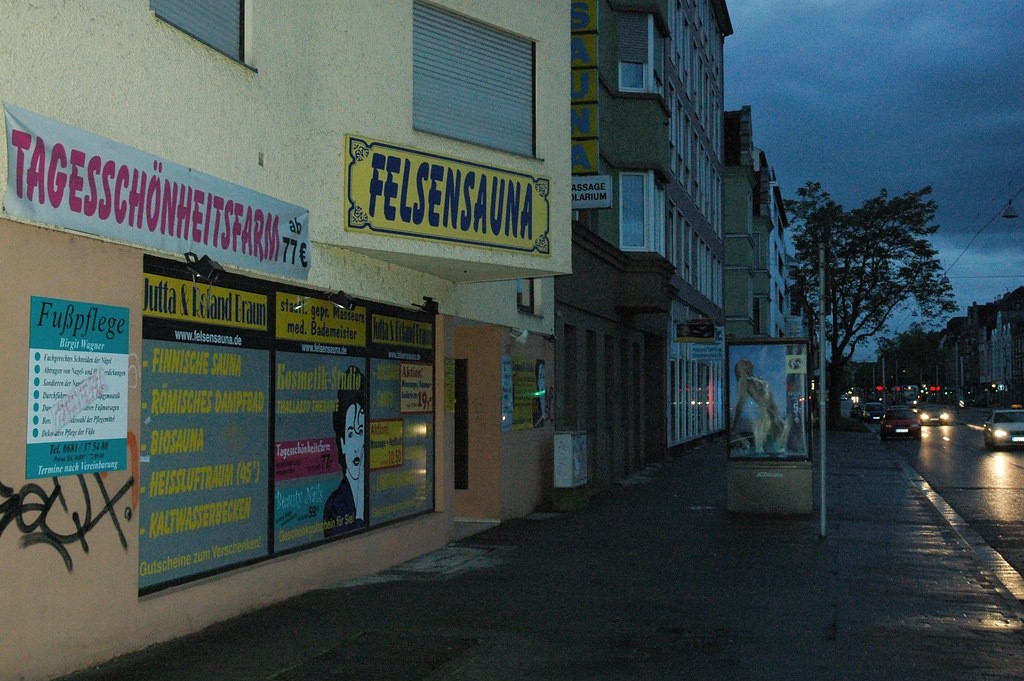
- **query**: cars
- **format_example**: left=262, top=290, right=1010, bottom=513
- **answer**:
left=880, top=406, right=922, bottom=441
left=983, top=404, right=1024, bottom=448
left=918, top=403, right=952, bottom=425
left=843, top=386, right=940, bottom=423
